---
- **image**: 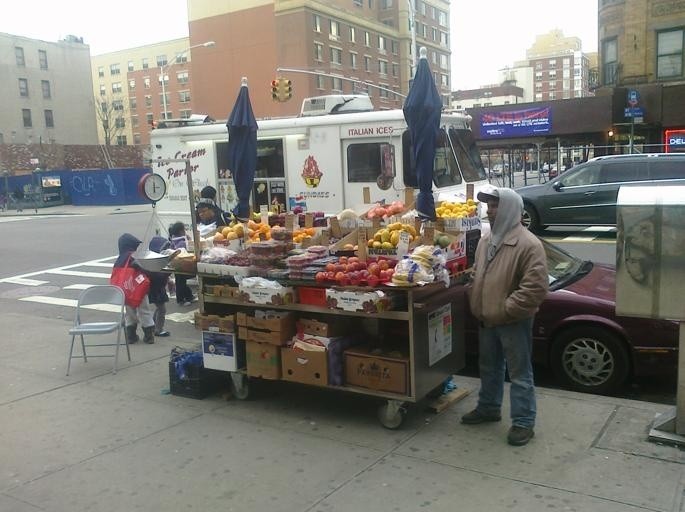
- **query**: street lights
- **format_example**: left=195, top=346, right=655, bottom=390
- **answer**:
left=160, top=41, right=215, bottom=127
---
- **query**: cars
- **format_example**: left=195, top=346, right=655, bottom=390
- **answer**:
left=464, top=236, right=679, bottom=395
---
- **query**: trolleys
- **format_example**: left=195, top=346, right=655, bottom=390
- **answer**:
left=196, top=201, right=482, bottom=429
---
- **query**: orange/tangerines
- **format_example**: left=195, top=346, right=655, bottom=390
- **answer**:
left=246, top=219, right=272, bottom=244
left=292, top=228, right=315, bottom=243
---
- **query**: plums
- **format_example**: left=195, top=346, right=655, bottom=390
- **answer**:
left=316, top=257, right=394, bottom=286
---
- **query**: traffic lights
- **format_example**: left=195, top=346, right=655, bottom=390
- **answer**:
left=284, top=80, right=292, bottom=100
left=271, top=79, right=280, bottom=103
left=607, top=128, right=616, bottom=136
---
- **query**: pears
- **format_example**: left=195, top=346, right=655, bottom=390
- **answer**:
left=214, top=223, right=245, bottom=243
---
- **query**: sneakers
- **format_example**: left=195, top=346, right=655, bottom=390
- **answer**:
left=180, top=298, right=192, bottom=308
left=188, top=295, right=200, bottom=303
left=461, top=408, right=503, bottom=424
left=506, top=425, right=535, bottom=446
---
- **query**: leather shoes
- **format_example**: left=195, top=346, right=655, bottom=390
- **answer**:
left=155, top=330, right=170, bottom=338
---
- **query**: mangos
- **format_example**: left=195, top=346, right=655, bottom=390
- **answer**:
left=368, top=220, right=417, bottom=250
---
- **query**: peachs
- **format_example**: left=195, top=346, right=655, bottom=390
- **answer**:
left=365, top=205, right=404, bottom=217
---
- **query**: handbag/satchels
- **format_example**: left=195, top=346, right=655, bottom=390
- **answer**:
left=111, top=253, right=151, bottom=308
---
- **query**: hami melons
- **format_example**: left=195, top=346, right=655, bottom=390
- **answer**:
left=433, top=233, right=450, bottom=248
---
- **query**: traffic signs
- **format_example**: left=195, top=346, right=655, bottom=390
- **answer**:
left=624, top=91, right=642, bottom=117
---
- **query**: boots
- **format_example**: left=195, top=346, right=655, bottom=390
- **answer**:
left=143, top=326, right=157, bottom=344
left=125, top=324, right=140, bottom=344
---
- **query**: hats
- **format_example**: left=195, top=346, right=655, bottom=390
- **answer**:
left=477, top=188, right=501, bottom=201
left=196, top=198, right=218, bottom=210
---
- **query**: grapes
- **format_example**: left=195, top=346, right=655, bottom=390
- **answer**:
left=225, top=254, right=252, bottom=267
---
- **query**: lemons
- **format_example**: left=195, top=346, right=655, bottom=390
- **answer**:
left=435, top=199, right=478, bottom=218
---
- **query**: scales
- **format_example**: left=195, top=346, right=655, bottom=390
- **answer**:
left=131, top=162, right=180, bottom=271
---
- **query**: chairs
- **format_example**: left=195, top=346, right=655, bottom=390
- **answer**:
left=64, top=283, right=132, bottom=378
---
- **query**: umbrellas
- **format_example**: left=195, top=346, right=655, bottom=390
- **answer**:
left=226, top=76, right=258, bottom=222
left=402, top=47, right=442, bottom=225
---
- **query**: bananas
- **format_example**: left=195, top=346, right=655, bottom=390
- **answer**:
left=392, top=247, right=441, bottom=285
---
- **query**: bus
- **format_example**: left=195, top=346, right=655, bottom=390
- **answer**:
left=150, top=95, right=497, bottom=294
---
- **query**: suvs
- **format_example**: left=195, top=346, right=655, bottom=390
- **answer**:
left=514, top=153, right=684, bottom=235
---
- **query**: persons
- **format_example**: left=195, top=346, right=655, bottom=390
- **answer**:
left=196, top=201, right=221, bottom=226
left=196, top=186, right=218, bottom=209
left=112, top=234, right=155, bottom=343
left=168, top=224, right=198, bottom=305
left=461, top=188, right=550, bottom=447
left=147, top=237, right=171, bottom=338
